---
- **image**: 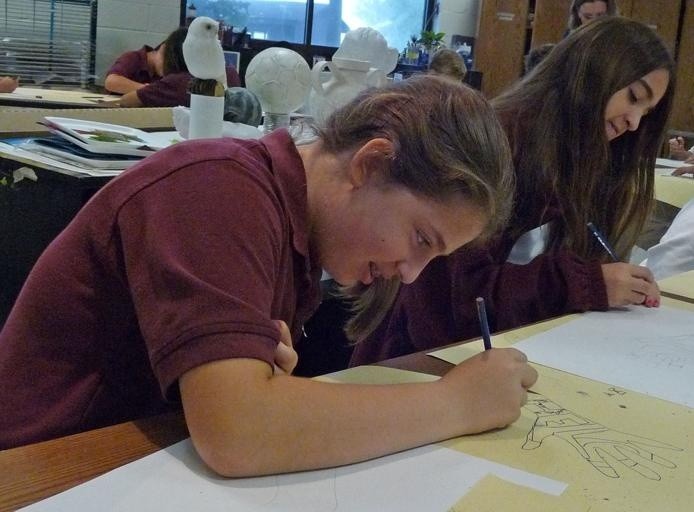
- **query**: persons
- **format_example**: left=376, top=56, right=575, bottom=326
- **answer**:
left=340, top=15, right=677, bottom=373
left=0, top=72, right=539, bottom=478
left=523, top=42, right=557, bottom=73
left=563, top=0, right=618, bottom=37
left=116, top=28, right=241, bottom=112
left=0, top=75, right=18, bottom=94
left=100, top=39, right=169, bottom=96
left=667, top=134, right=694, bottom=180
left=429, top=48, right=466, bottom=86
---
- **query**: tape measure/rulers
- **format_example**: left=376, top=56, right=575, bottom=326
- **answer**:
left=660, top=291, right=694, bottom=304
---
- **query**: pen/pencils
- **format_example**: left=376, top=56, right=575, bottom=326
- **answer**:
left=587, top=223, right=619, bottom=263
left=82, top=97, right=103, bottom=98
left=476, top=296, right=492, bottom=350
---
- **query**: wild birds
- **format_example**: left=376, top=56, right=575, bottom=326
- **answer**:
left=179, top=16, right=230, bottom=91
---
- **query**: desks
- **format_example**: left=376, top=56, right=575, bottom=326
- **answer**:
left=0, top=88, right=694, bottom=512
